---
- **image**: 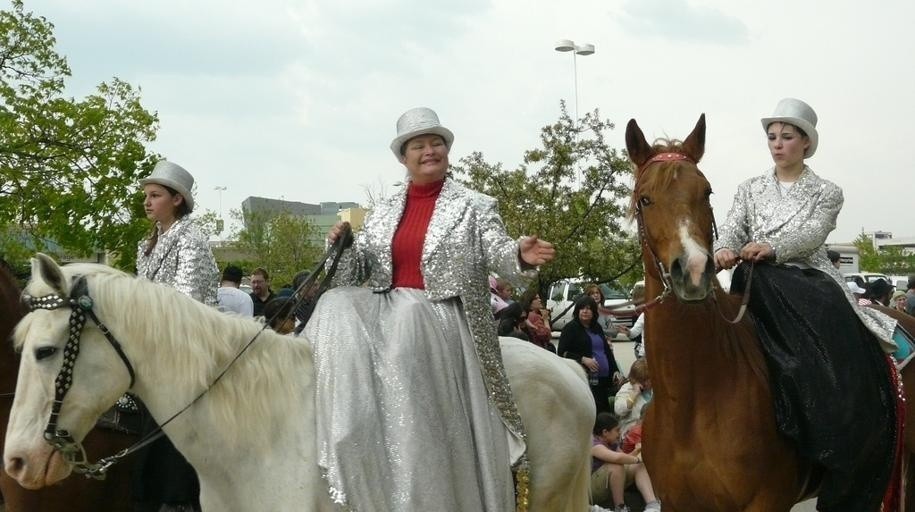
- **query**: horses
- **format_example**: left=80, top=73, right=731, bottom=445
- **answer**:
left=3, top=252, right=596, bottom=512
left=0, top=259, right=140, bottom=512
left=625, top=113, right=915, bottom=512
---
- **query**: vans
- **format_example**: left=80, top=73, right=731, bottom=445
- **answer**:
left=546, top=278, right=634, bottom=340
left=843, top=272, right=895, bottom=299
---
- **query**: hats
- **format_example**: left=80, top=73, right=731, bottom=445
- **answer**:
left=762, top=99, right=818, bottom=158
left=391, top=108, right=454, bottom=167
left=138, top=160, right=194, bottom=212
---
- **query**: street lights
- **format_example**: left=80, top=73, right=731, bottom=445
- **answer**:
left=554, top=39, right=596, bottom=189
left=212, top=185, right=228, bottom=218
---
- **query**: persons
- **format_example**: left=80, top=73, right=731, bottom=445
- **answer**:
left=303, top=107, right=557, bottom=512
left=489, top=270, right=661, bottom=511
left=827, top=251, right=915, bottom=316
left=136, top=160, right=223, bottom=309
left=216, top=266, right=319, bottom=337
left=715, top=98, right=898, bottom=511
